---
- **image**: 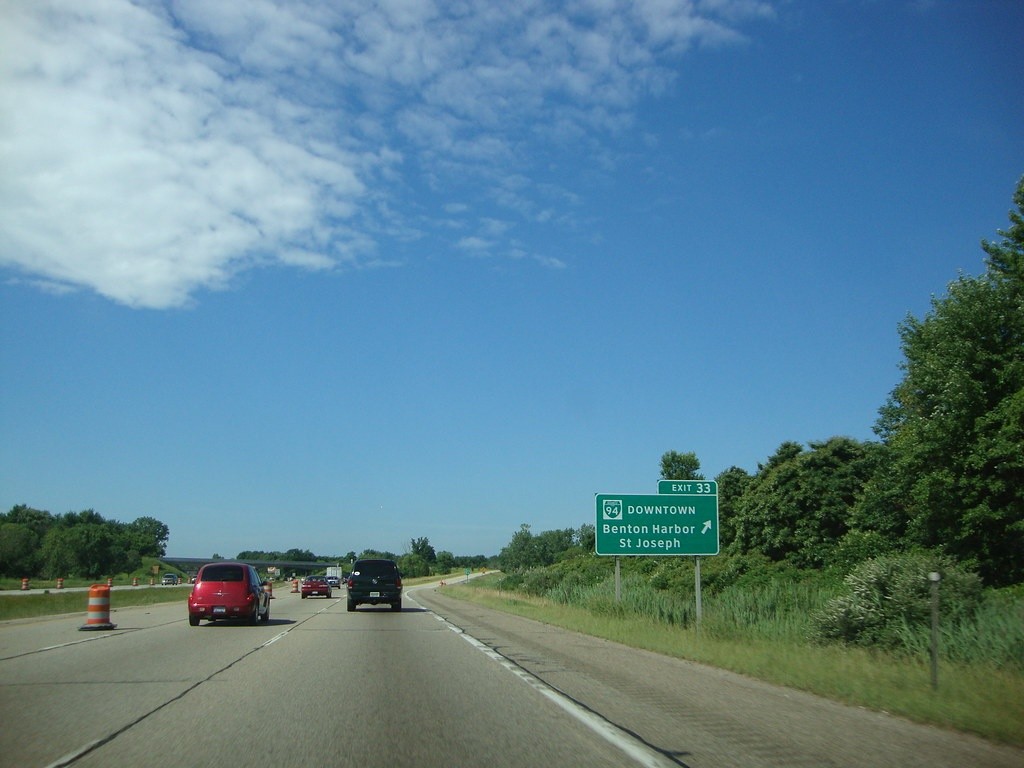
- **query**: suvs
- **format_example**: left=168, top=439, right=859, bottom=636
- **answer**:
left=345, top=558, right=402, bottom=611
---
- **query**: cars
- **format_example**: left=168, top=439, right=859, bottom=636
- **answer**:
left=325, top=575, right=340, bottom=587
left=192, top=575, right=197, bottom=582
left=187, top=562, right=271, bottom=625
left=162, top=574, right=178, bottom=584
left=302, top=574, right=332, bottom=598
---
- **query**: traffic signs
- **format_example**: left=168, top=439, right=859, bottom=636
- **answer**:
left=593, top=480, right=717, bottom=553
